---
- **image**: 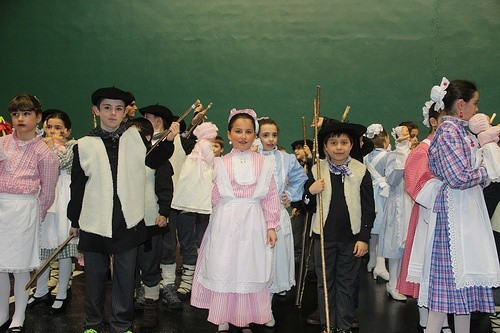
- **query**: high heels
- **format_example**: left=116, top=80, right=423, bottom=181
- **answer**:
left=6, top=308, right=35, bottom=333
left=440, top=326, right=455, bottom=333
left=27, top=291, right=51, bottom=307
left=385, top=281, right=408, bottom=301
left=418, top=323, right=427, bottom=333
left=48, top=296, right=69, bottom=313
left=0, top=318, right=11, bottom=333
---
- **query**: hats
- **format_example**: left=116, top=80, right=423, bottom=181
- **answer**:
left=138, top=105, right=174, bottom=124
left=91, top=86, right=134, bottom=107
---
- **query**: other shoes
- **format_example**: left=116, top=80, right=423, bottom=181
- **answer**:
left=307, top=309, right=320, bottom=325
left=140, top=298, right=160, bottom=309
left=161, top=285, right=183, bottom=310
left=218, top=322, right=229, bottom=331
left=265, top=314, right=275, bottom=327
left=82, top=326, right=102, bottom=333
left=121, top=327, right=135, bottom=333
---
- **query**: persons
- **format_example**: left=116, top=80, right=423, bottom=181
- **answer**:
left=20, top=109, right=79, bottom=316
left=0, top=93, right=59, bottom=333
left=66, top=78, right=500, bottom=333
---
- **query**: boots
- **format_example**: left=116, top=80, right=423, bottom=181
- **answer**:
left=48, top=261, right=60, bottom=289
left=373, top=246, right=389, bottom=280
left=51, top=262, right=76, bottom=296
left=367, top=238, right=377, bottom=272
left=177, top=264, right=196, bottom=295
